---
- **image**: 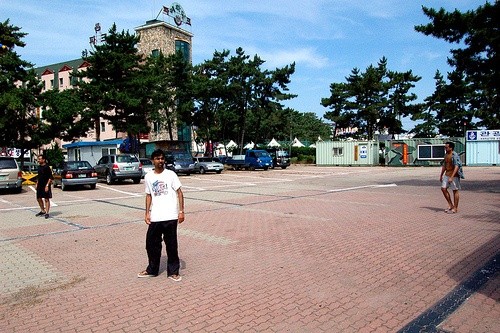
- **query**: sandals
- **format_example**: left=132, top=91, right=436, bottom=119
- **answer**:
left=444, top=207, right=451, bottom=212
left=169, top=274, right=182, bottom=282
left=447, top=209, right=455, bottom=214
left=137, top=271, right=155, bottom=277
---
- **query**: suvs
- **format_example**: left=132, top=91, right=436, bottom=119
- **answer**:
left=164, top=151, right=195, bottom=175
left=92, top=154, right=143, bottom=185
left=0, top=156, right=23, bottom=189
left=268, top=150, right=291, bottom=169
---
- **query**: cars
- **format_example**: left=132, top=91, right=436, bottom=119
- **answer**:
left=52, top=161, right=99, bottom=191
left=193, top=157, right=224, bottom=174
left=139, top=158, right=155, bottom=179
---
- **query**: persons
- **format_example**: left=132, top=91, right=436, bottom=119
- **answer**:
left=36, top=154, right=53, bottom=219
left=137, top=149, right=185, bottom=281
left=439, top=142, right=462, bottom=214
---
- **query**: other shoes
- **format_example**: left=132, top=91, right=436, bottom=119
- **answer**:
left=35, top=211, right=46, bottom=216
left=45, top=214, right=49, bottom=219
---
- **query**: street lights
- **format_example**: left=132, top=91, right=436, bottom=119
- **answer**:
left=427, top=101, right=441, bottom=138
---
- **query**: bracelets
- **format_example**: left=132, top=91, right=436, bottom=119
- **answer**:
left=46, top=183, right=49, bottom=187
left=179, top=211, right=184, bottom=214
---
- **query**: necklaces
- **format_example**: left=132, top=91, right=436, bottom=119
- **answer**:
left=381, top=147, right=386, bottom=167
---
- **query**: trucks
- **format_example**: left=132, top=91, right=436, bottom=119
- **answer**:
left=226, top=149, right=273, bottom=172
